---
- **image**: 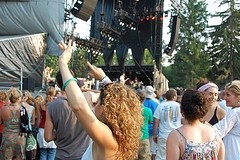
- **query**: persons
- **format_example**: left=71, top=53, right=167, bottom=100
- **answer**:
left=196, top=80, right=240, bottom=160
left=119, top=73, right=188, bottom=160
left=57, top=38, right=143, bottom=160
left=0, top=65, right=96, bottom=160
left=165, top=90, right=225, bottom=160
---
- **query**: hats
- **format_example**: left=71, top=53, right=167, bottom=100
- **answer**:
left=142, top=86, right=157, bottom=100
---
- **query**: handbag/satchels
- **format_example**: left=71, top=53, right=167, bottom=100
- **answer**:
left=32, top=124, right=38, bottom=140
left=18, top=111, right=32, bottom=138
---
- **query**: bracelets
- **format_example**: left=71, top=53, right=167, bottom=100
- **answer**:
left=99, top=75, right=109, bottom=82
left=62, top=78, right=78, bottom=90
